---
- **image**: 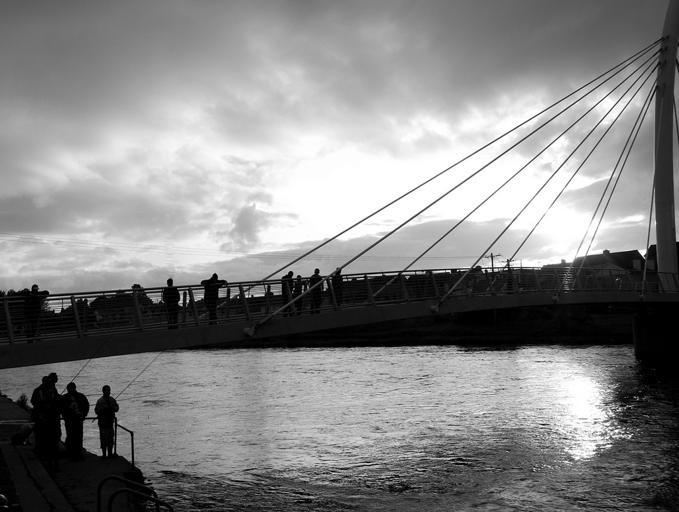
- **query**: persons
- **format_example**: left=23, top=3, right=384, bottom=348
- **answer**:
left=163, top=278, right=180, bottom=329
left=200, top=273, right=226, bottom=325
left=309, top=269, right=324, bottom=315
left=20, top=284, right=49, bottom=344
left=29, top=373, right=90, bottom=461
left=94, top=385, right=119, bottom=457
left=281, top=271, right=294, bottom=317
left=293, top=275, right=307, bottom=317
left=332, top=268, right=344, bottom=304
